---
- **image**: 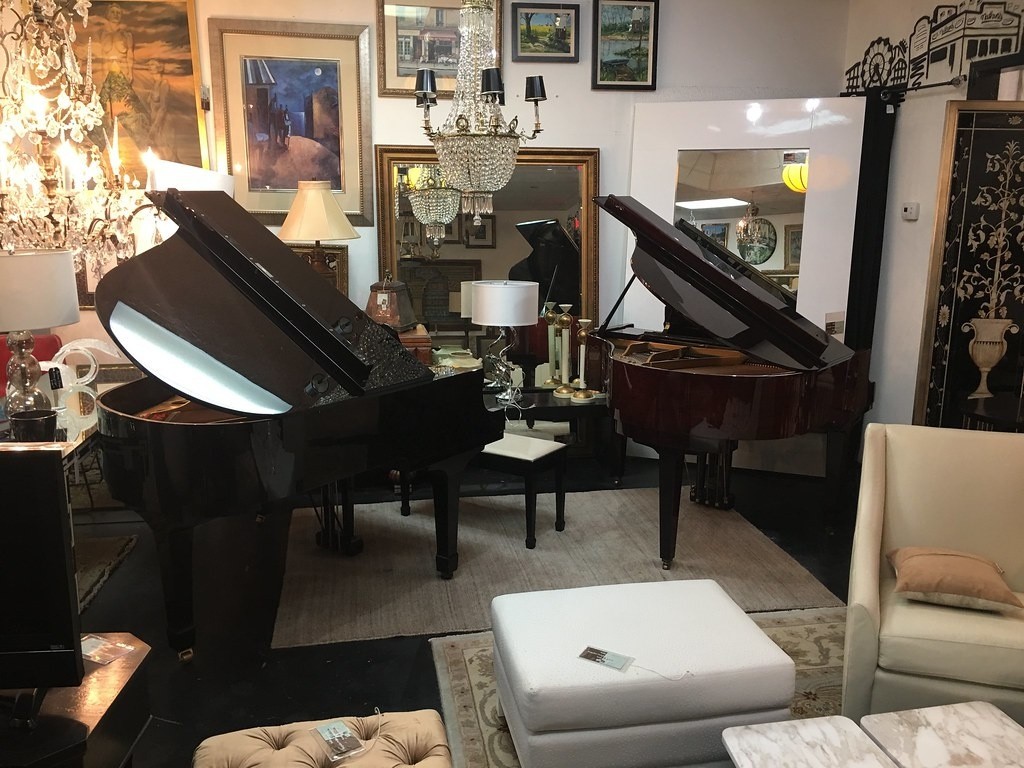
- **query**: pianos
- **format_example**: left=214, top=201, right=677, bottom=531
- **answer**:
left=95, top=188, right=509, bottom=663
left=505, top=218, right=583, bottom=430
left=582, top=193, right=875, bottom=571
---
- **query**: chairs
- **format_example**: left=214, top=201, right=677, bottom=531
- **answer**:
left=0, top=334, right=103, bottom=511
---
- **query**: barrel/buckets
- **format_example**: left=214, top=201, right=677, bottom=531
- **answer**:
left=10, top=409, right=58, bottom=442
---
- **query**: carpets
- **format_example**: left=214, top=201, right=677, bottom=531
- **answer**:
left=428, top=608, right=845, bottom=766
left=270, top=482, right=846, bottom=650
left=76, top=533, right=140, bottom=615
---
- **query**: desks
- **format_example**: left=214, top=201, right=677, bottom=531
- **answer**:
left=469, top=391, right=627, bottom=488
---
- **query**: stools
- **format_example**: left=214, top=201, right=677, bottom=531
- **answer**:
left=192, top=709, right=452, bottom=768
left=490, top=578, right=797, bottom=767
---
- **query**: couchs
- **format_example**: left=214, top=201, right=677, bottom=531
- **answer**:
left=838, top=423, right=1024, bottom=720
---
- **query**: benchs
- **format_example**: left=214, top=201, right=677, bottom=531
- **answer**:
left=397, top=433, right=571, bottom=550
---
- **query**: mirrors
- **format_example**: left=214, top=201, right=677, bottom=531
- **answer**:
left=375, top=144, right=602, bottom=390
left=664, top=148, right=810, bottom=313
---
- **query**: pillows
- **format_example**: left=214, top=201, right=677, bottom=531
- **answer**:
left=885, top=548, right=1023, bottom=615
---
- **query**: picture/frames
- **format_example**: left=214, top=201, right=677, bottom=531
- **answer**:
left=442, top=213, right=462, bottom=244
left=285, top=242, right=349, bottom=300
left=399, top=211, right=422, bottom=244
left=395, top=257, right=482, bottom=331
left=207, top=17, right=375, bottom=228
left=465, top=213, right=497, bottom=250
left=761, top=269, right=799, bottom=298
left=701, top=222, right=729, bottom=249
left=590, top=0, right=659, bottom=90
left=376, top=0, right=504, bottom=99
left=783, top=224, right=803, bottom=268
left=20, top=0, right=210, bottom=196
left=910, top=101, right=1024, bottom=432
left=511, top=2, right=580, bottom=64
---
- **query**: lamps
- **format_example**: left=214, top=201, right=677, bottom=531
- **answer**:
left=413, top=0, right=549, bottom=228
left=782, top=163, right=808, bottom=193
left=471, top=280, right=540, bottom=402
left=394, top=163, right=463, bottom=247
left=459, top=280, right=510, bottom=388
left=0, top=249, right=81, bottom=437
left=735, top=191, right=766, bottom=243
left=278, top=180, right=361, bottom=290
left=0, top=0, right=170, bottom=282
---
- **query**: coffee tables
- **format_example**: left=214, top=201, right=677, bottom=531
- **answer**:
left=721, top=702, right=1024, bottom=768
